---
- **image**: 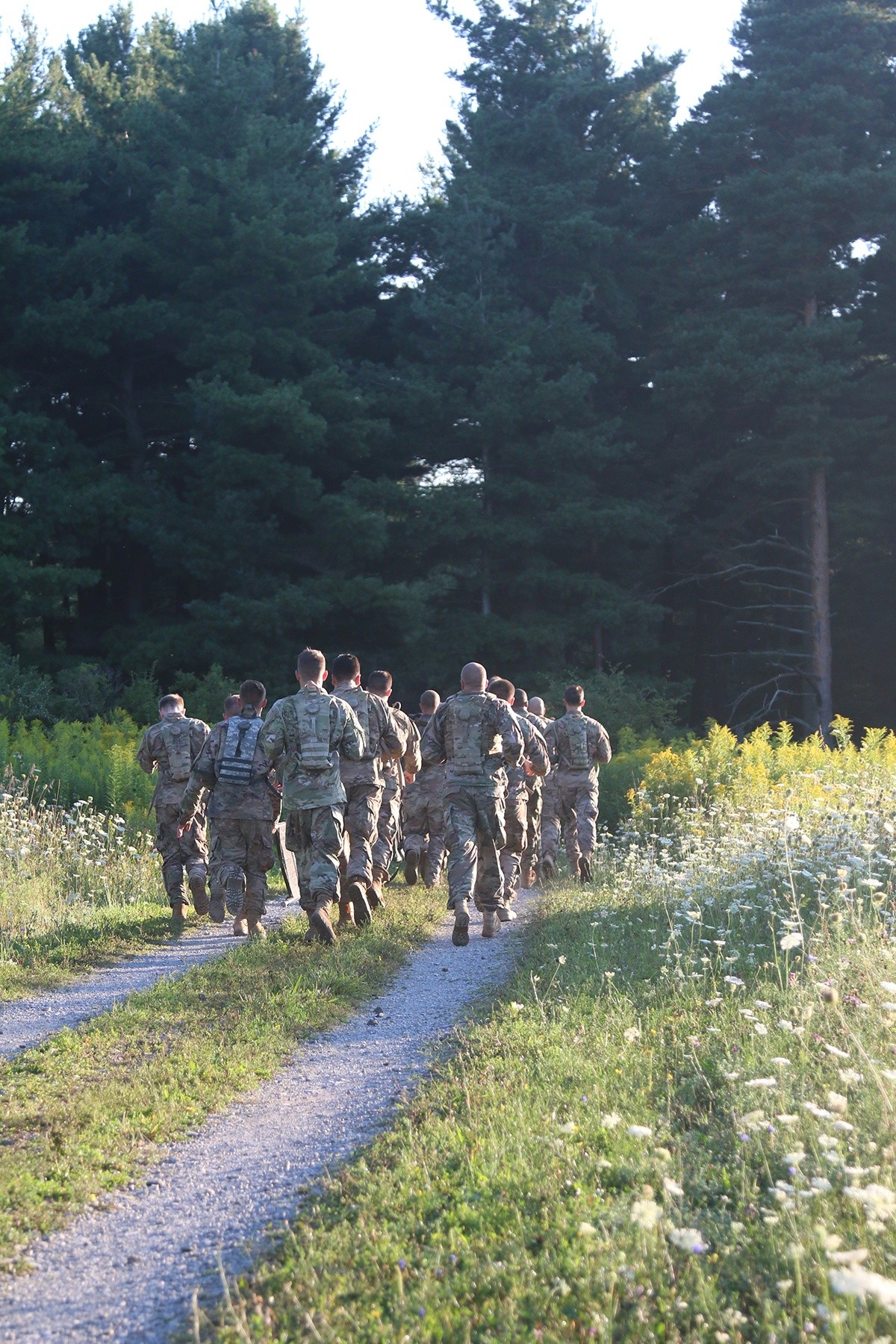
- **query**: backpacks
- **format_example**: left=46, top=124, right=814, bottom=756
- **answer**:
left=560, top=715, right=593, bottom=770
left=332, top=685, right=382, bottom=759
left=449, top=692, right=497, bottom=776
left=290, top=680, right=338, bottom=770
left=217, top=703, right=264, bottom=786
left=160, top=717, right=191, bottom=782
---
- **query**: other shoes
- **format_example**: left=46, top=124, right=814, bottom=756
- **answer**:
left=404, top=849, right=418, bottom=885
left=419, top=848, right=429, bottom=880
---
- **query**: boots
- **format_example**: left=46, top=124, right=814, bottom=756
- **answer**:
left=541, top=852, right=557, bottom=880
left=569, top=862, right=580, bottom=881
left=438, top=869, right=446, bottom=885
left=497, top=894, right=517, bottom=922
left=578, top=849, right=593, bottom=883
left=481, top=908, right=501, bottom=936
left=451, top=899, right=471, bottom=946
left=165, top=867, right=267, bottom=941
left=521, top=867, right=537, bottom=890
left=536, top=865, right=545, bottom=881
left=514, top=876, right=520, bottom=889
left=301, top=866, right=387, bottom=946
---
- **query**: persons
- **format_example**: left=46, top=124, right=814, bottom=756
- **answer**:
left=258, top=647, right=367, bottom=946
left=329, top=654, right=409, bottom=932
left=139, top=692, right=210, bottom=940
left=179, top=670, right=561, bottom=947
left=419, top=661, right=525, bottom=947
left=542, top=685, right=612, bottom=884
